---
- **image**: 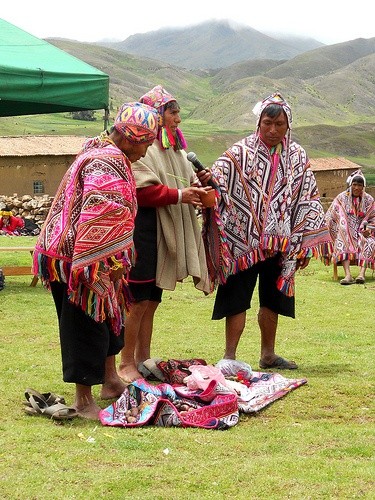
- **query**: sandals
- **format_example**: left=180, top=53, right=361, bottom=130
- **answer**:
left=22, top=395, right=77, bottom=420
left=20, top=388, right=69, bottom=409
left=259, top=355, right=297, bottom=369
left=355, top=275, right=367, bottom=284
left=341, top=276, right=355, bottom=285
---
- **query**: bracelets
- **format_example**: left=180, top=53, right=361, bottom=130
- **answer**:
left=176, top=188, right=183, bottom=206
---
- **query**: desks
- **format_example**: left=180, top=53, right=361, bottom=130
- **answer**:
left=0, top=247, right=39, bottom=287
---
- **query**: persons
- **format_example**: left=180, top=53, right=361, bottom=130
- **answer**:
left=0, top=208, right=41, bottom=236
left=194, top=88, right=333, bottom=369
left=116, top=83, right=219, bottom=384
left=31, top=101, right=159, bottom=421
left=318, top=169, right=375, bottom=285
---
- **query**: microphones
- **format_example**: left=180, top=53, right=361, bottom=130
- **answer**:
left=187, top=152, right=221, bottom=195
left=362, top=221, right=368, bottom=230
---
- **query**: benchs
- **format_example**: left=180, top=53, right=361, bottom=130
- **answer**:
left=0, top=266, right=39, bottom=287
